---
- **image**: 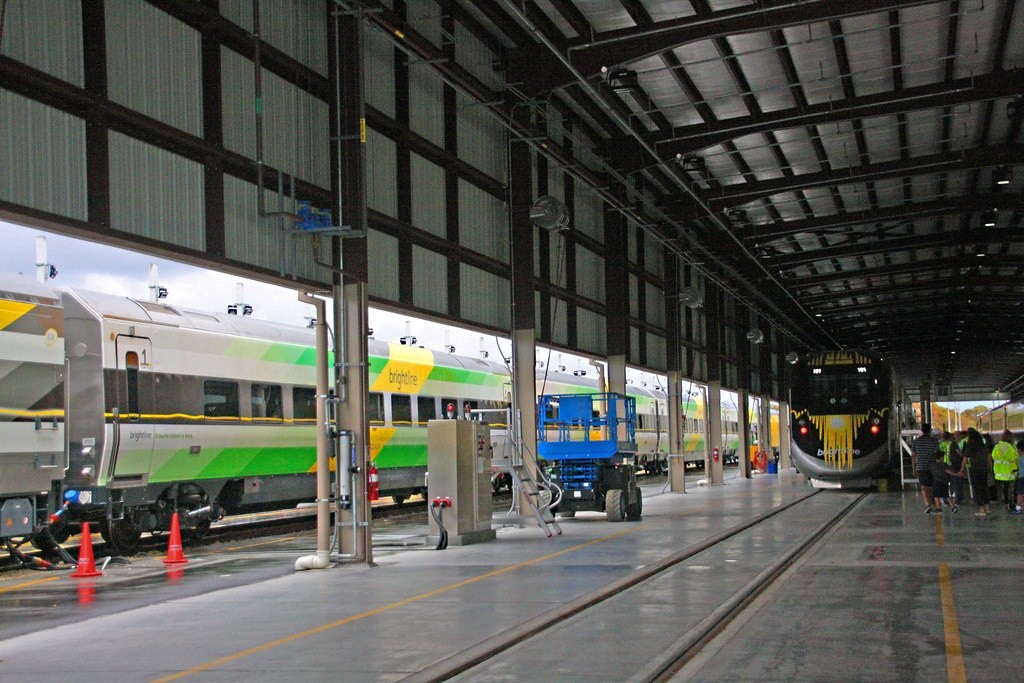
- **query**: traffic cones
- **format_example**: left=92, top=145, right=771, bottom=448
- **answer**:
left=71, top=522, right=102, bottom=577
left=163, top=513, right=188, bottom=563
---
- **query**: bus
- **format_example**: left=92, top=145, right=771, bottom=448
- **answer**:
left=791, top=348, right=916, bottom=489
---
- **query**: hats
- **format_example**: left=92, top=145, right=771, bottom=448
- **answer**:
left=931, top=429, right=943, bottom=435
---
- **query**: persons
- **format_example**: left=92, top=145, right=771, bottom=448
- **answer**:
left=939, top=430, right=998, bottom=508
left=1014, top=441, right=1024, bottom=512
left=992, top=430, right=1021, bottom=513
left=911, top=423, right=939, bottom=513
left=959, top=427, right=993, bottom=516
left=930, top=451, right=961, bottom=514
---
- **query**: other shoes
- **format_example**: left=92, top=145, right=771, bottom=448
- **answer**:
left=1009, top=509, right=1022, bottom=514
left=924, top=505, right=932, bottom=513
left=931, top=508, right=942, bottom=514
left=949, top=505, right=958, bottom=514
left=975, top=508, right=990, bottom=516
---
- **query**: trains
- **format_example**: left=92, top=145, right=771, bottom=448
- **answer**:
left=0, top=282, right=790, bottom=571
